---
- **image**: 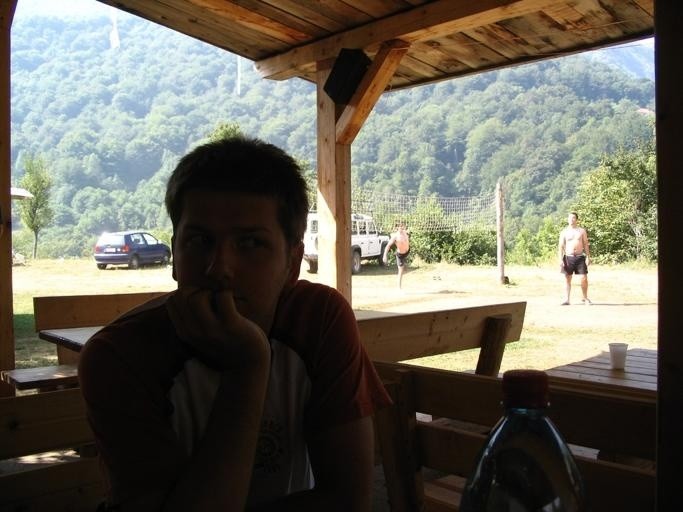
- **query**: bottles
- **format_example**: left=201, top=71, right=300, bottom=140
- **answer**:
left=458, top=369, right=596, bottom=512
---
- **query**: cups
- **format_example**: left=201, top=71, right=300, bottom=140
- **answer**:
left=608, top=343, right=628, bottom=370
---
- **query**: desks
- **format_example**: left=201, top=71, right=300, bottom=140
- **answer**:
left=543, top=347, right=657, bottom=401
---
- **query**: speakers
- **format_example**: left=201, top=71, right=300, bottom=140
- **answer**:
left=323, top=46, right=372, bottom=106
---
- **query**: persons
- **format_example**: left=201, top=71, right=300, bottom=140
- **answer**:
left=559, top=213, right=592, bottom=305
left=382, top=224, right=410, bottom=287
left=75, top=136, right=392, bottom=509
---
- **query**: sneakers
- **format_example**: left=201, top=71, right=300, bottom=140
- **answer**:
left=581, top=297, right=592, bottom=305
left=562, top=301, right=570, bottom=305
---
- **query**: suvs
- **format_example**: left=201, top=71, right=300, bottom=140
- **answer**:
left=303, top=213, right=390, bottom=275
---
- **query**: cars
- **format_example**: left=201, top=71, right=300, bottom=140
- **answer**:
left=94, top=230, right=171, bottom=270
